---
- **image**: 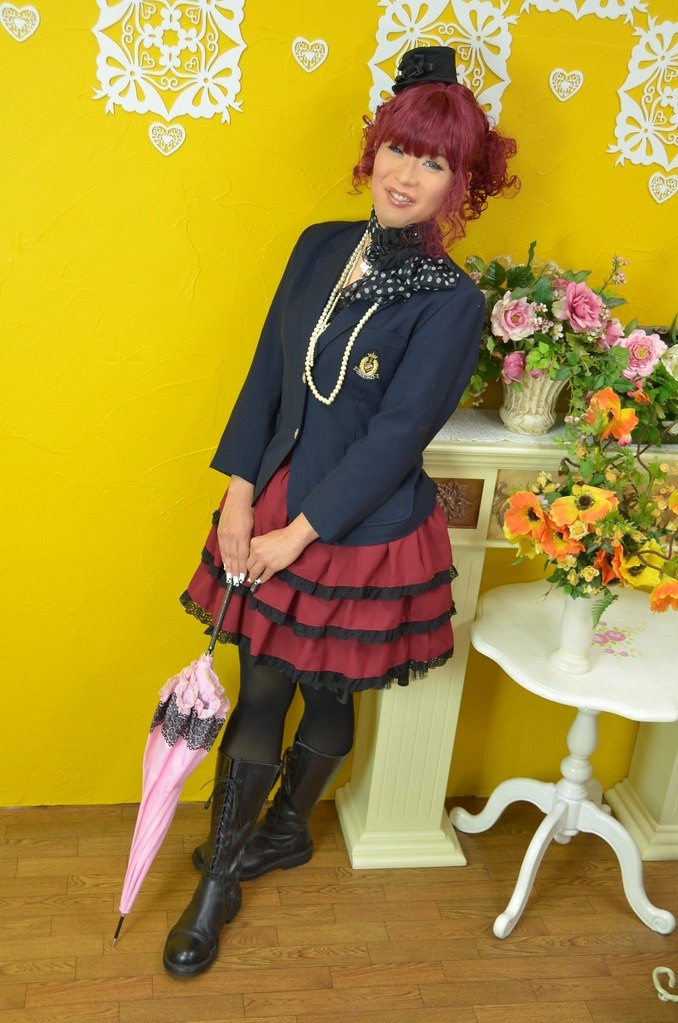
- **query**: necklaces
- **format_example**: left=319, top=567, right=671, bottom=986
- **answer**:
left=304, top=230, right=383, bottom=405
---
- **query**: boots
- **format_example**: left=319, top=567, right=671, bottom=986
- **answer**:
left=192, top=733, right=347, bottom=879
left=164, top=745, right=286, bottom=978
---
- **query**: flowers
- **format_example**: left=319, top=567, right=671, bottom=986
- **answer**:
left=461, top=236, right=667, bottom=423
left=500, top=386, right=677, bottom=628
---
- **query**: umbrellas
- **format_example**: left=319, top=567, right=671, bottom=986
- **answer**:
left=112, top=585, right=231, bottom=946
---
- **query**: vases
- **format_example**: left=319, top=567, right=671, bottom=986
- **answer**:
left=500, top=366, right=569, bottom=432
left=550, top=585, right=623, bottom=674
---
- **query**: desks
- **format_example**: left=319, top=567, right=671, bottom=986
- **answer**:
left=449, top=578, right=678, bottom=940
left=330, top=406, right=678, bottom=871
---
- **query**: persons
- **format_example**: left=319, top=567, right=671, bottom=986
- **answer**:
left=163, top=46, right=520, bottom=977
